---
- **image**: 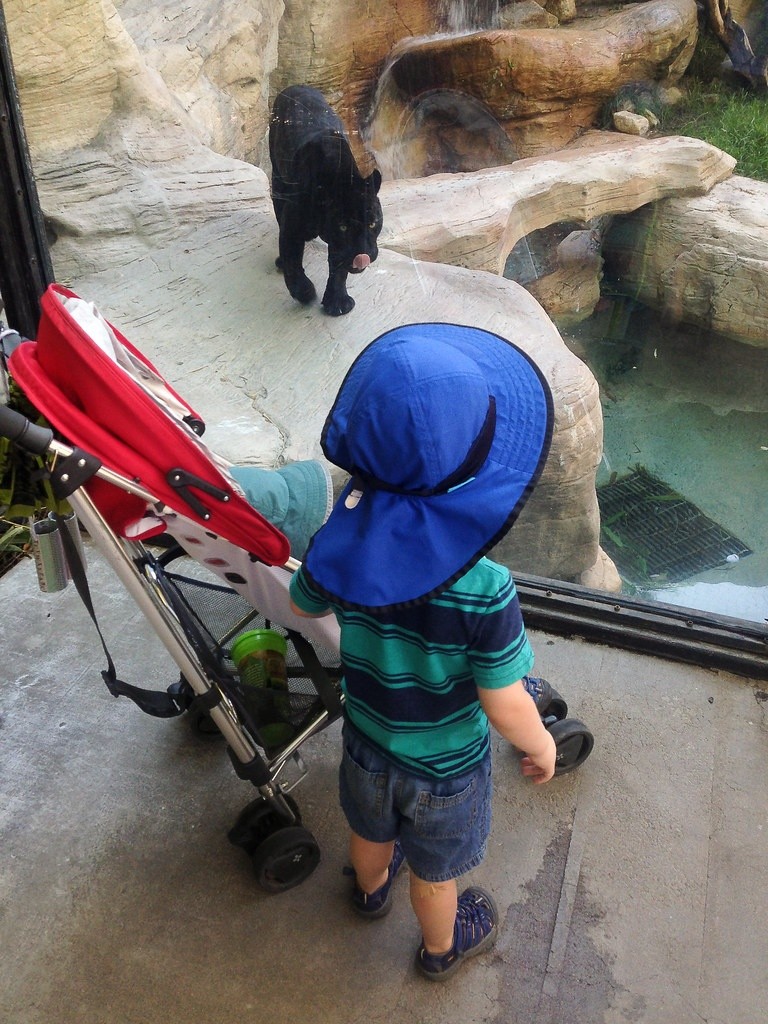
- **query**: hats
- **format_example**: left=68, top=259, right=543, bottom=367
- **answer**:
left=302, top=324, right=554, bottom=614
left=230, top=459, right=333, bottom=563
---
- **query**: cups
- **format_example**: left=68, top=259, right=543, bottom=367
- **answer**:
left=231, top=629, right=290, bottom=750
left=48, top=509, right=87, bottom=581
left=34, top=519, right=67, bottom=593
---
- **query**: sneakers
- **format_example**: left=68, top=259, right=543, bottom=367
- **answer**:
left=341, top=836, right=405, bottom=919
left=520, top=674, right=553, bottom=714
left=416, top=886, right=499, bottom=982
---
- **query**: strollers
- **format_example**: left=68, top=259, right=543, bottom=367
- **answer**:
left=0, top=282, right=594, bottom=895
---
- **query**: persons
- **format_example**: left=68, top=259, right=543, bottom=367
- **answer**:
left=288, top=324, right=558, bottom=984
left=225, top=459, right=552, bottom=713
